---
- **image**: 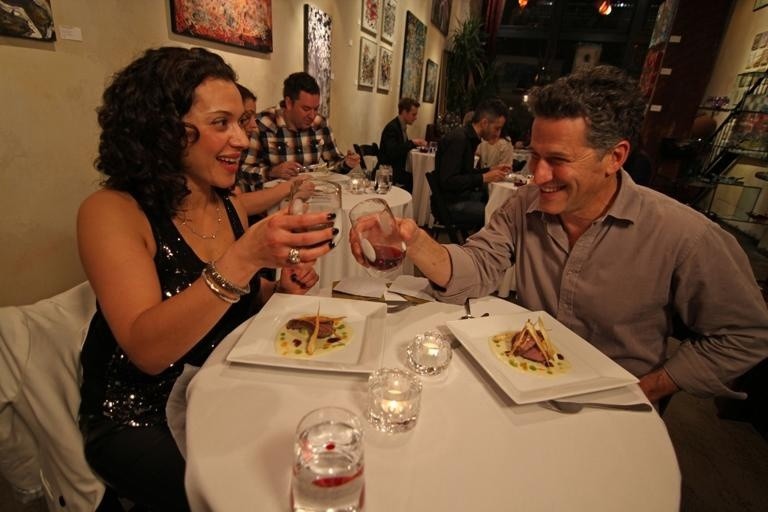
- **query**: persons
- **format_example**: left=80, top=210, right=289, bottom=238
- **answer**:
left=476, top=108, right=516, bottom=206
left=77, top=45, right=339, bottom=512
left=233, top=82, right=318, bottom=227
left=238, top=71, right=361, bottom=185
left=368, top=96, right=427, bottom=193
left=349, top=61, right=768, bottom=416
left=429, top=98, right=510, bottom=231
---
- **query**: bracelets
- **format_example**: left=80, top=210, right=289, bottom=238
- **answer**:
left=272, top=280, right=280, bottom=293
left=201, top=258, right=251, bottom=304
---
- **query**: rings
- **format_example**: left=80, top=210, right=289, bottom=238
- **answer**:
left=289, top=247, right=301, bottom=264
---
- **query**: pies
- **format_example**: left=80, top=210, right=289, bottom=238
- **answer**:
left=490, top=330, right=567, bottom=376
left=276, top=316, right=352, bottom=358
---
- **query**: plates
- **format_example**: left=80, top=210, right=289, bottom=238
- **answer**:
left=445, top=308, right=642, bottom=406
left=224, top=291, right=388, bottom=374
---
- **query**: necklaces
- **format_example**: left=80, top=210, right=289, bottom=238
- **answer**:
left=173, top=195, right=224, bottom=240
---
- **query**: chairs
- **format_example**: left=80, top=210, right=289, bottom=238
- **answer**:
left=1, top=281, right=106, bottom=512
left=425, top=170, right=483, bottom=244
left=354, top=143, right=380, bottom=169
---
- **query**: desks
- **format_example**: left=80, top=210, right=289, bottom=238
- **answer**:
left=263, top=171, right=414, bottom=281
left=409, top=145, right=480, bottom=226
left=184, top=275, right=681, bottom=512
left=485, top=178, right=531, bottom=226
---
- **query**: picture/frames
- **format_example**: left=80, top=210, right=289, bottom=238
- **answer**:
left=753, top=1, right=768, bottom=13
left=357, top=1, right=474, bottom=123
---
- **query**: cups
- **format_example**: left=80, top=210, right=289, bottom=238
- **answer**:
left=414, top=140, right=438, bottom=154
left=291, top=164, right=409, bottom=272
left=505, top=170, right=530, bottom=186
left=280, top=331, right=452, bottom=511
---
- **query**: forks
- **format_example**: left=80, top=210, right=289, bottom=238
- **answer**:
left=549, top=400, right=654, bottom=415
